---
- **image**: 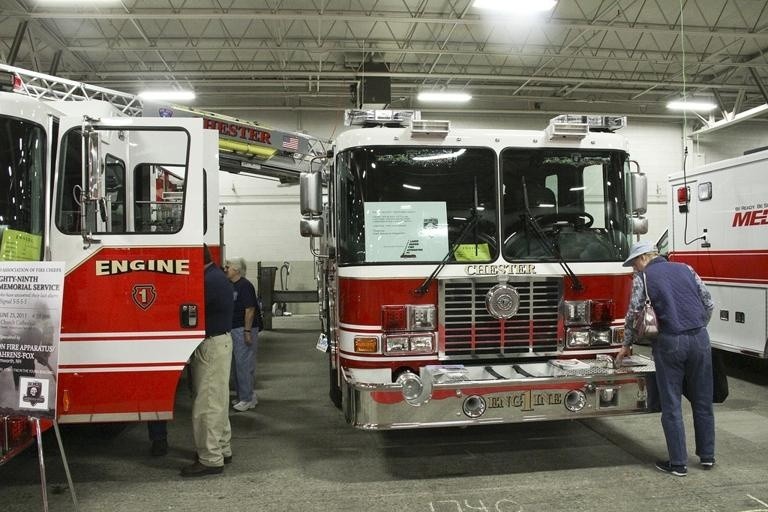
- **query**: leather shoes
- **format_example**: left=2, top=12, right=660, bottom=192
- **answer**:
left=179, top=462, right=223, bottom=477
left=151, top=440, right=168, bottom=456
left=192, top=452, right=233, bottom=463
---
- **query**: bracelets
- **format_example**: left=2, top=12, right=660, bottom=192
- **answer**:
left=243, top=329, right=251, bottom=332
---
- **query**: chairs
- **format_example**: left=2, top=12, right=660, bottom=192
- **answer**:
left=504, top=183, right=557, bottom=238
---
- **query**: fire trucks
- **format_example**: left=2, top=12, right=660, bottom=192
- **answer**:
left=299, top=108, right=661, bottom=433
left=0, top=62, right=334, bottom=465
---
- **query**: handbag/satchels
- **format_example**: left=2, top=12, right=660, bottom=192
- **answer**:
left=633, top=271, right=658, bottom=339
left=683, top=348, right=729, bottom=404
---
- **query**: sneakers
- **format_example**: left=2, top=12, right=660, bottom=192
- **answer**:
left=655, top=461, right=687, bottom=477
left=232, top=392, right=258, bottom=405
left=233, top=399, right=256, bottom=412
left=700, top=458, right=714, bottom=466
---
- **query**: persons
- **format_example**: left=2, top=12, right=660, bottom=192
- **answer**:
left=73, top=418, right=170, bottom=458
left=222, top=257, right=263, bottom=412
left=178, top=244, right=235, bottom=476
left=613, top=241, right=715, bottom=476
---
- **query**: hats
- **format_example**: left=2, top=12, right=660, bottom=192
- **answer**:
left=622, top=241, right=659, bottom=267
